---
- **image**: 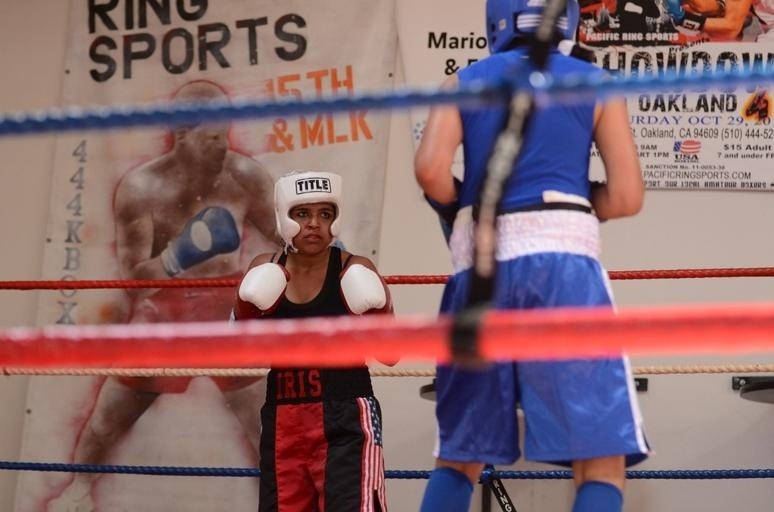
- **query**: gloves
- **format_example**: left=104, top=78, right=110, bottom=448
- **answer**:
left=232, top=261, right=291, bottom=321
left=339, top=263, right=395, bottom=317
left=161, top=206, right=240, bottom=277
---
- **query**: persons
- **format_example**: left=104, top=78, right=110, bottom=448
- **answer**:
left=414, top=0, right=648, bottom=512
left=42, top=80, right=297, bottom=512
left=221, top=169, right=397, bottom=511
left=658, top=2, right=774, bottom=44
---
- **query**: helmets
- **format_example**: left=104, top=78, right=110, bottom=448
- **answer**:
left=486, top=0, right=580, bottom=57
left=274, top=168, right=344, bottom=254
